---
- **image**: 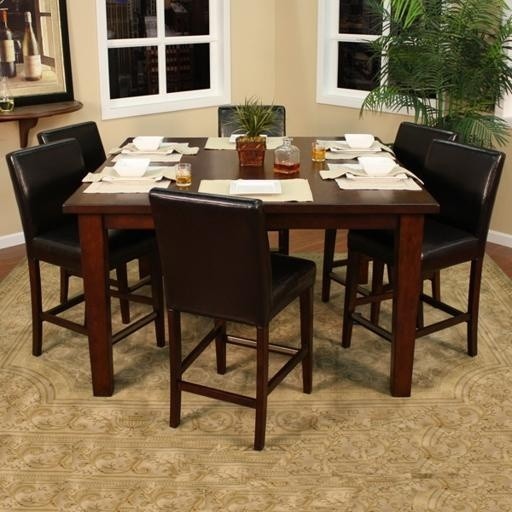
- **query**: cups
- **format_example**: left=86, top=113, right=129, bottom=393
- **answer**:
left=176, top=163, right=192, bottom=187
left=312, top=142, right=326, bottom=163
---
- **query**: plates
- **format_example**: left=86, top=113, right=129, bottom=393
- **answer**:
left=229, top=180, right=282, bottom=195
left=328, top=141, right=382, bottom=153
left=122, top=143, right=178, bottom=155
left=100, top=166, right=164, bottom=183
left=343, top=163, right=408, bottom=181
left=229, top=134, right=267, bottom=144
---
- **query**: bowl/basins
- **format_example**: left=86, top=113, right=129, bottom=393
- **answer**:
left=113, top=159, right=149, bottom=178
left=344, top=134, right=375, bottom=149
left=132, top=136, right=163, bottom=151
left=357, top=157, right=396, bottom=175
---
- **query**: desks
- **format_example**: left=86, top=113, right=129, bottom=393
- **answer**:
left=1, top=100, right=82, bottom=147
left=63, top=134, right=440, bottom=398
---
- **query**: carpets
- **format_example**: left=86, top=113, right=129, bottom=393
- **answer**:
left=0, top=244, right=511, bottom=511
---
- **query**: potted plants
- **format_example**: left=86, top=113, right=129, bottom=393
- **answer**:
left=218, top=95, right=284, bottom=168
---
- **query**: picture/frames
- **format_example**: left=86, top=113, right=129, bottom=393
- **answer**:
left=1, top=0, right=73, bottom=106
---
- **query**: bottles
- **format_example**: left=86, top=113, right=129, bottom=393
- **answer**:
left=1, top=8, right=16, bottom=77
left=0, top=74, right=16, bottom=111
left=23, top=12, right=43, bottom=81
left=273, top=137, right=300, bottom=175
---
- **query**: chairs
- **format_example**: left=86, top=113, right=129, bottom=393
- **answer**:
left=146, top=189, right=314, bottom=451
left=340, top=138, right=506, bottom=358
left=218, top=100, right=292, bottom=257
left=38, top=120, right=148, bottom=323
left=6, top=138, right=167, bottom=355
left=318, top=122, right=459, bottom=326
